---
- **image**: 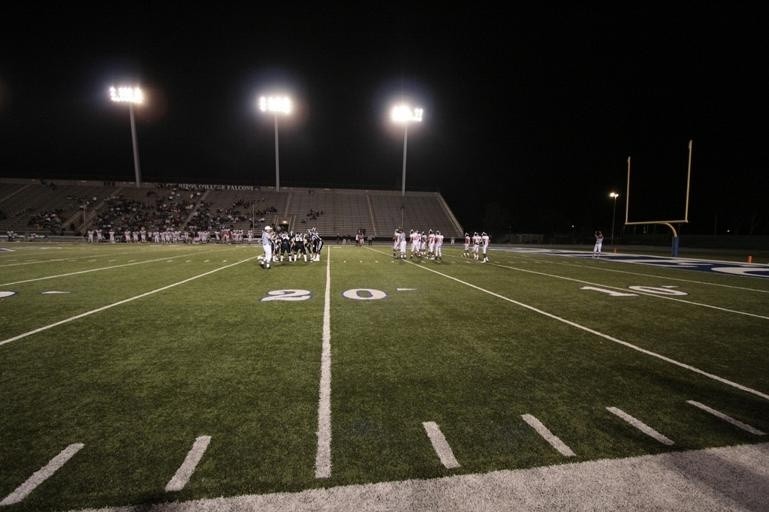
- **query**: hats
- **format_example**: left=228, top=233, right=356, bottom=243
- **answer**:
left=264, top=225, right=272, bottom=230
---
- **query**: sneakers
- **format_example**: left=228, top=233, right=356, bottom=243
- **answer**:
left=313, top=258, right=320, bottom=261
left=259, top=263, right=264, bottom=269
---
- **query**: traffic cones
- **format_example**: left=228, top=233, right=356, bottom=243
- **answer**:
left=612, top=248, right=617, bottom=255
left=746, top=255, right=752, bottom=265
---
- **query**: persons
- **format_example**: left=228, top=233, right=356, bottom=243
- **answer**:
left=592, top=229, right=604, bottom=259
left=391, top=226, right=491, bottom=264
left=83, top=185, right=205, bottom=247
left=303, top=208, right=372, bottom=246
left=29, top=180, right=96, bottom=228
left=259, top=226, right=324, bottom=268
left=184, top=199, right=269, bottom=244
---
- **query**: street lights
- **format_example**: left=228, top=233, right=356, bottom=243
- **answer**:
left=389, top=102, right=424, bottom=196
left=108, top=81, right=149, bottom=189
left=607, top=192, right=621, bottom=245
left=259, top=92, right=294, bottom=193
